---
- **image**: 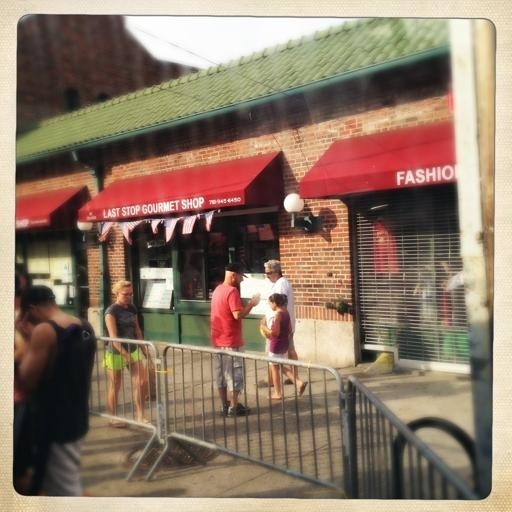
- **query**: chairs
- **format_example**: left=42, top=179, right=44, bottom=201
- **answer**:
left=223, top=399, right=249, bottom=416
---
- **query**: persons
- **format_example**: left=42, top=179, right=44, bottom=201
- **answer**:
left=103, top=281, right=155, bottom=428
left=209, top=262, right=260, bottom=416
left=260, top=293, right=308, bottom=400
left=14, top=264, right=97, bottom=497
left=256, top=259, right=299, bottom=388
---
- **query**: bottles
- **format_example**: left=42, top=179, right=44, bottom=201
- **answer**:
left=225, top=263, right=249, bottom=279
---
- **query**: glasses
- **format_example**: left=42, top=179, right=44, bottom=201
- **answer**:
left=76, top=216, right=96, bottom=243
left=283, top=192, right=317, bottom=231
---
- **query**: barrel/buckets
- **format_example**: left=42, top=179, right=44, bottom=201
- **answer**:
left=108, top=420, right=128, bottom=427
left=256, top=379, right=307, bottom=400
